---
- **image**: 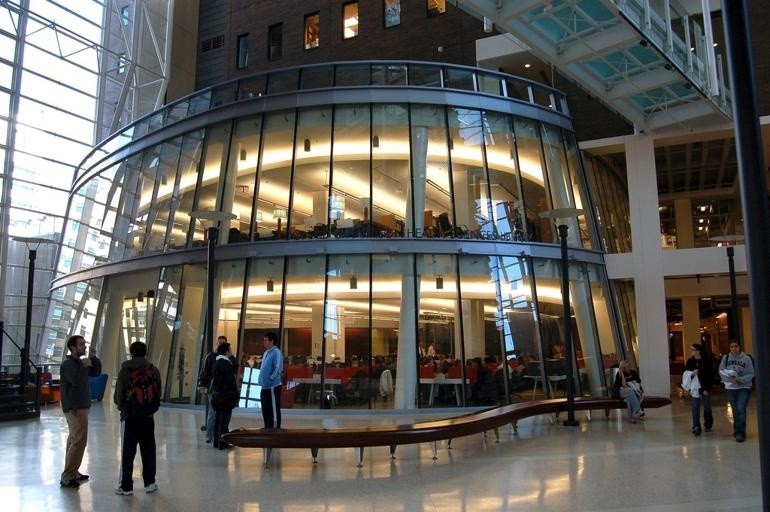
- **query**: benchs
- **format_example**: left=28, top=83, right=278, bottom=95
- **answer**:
left=219, top=393, right=675, bottom=470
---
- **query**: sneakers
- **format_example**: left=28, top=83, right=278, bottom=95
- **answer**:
left=144, top=483, right=160, bottom=494
left=74, top=470, right=90, bottom=481
left=60, top=478, right=80, bottom=487
left=114, top=485, right=133, bottom=496
left=691, top=424, right=746, bottom=442
left=200, top=425, right=234, bottom=450
left=628, top=412, right=646, bottom=425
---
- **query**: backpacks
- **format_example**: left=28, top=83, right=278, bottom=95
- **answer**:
left=122, top=362, right=156, bottom=412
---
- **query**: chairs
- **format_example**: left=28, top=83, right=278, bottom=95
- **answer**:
left=202, top=211, right=461, bottom=244
left=15, top=369, right=108, bottom=409
left=275, top=354, right=623, bottom=408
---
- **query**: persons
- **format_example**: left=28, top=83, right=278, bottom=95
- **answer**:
left=510, top=209, right=524, bottom=242
left=41, top=366, right=52, bottom=384
left=684, top=344, right=713, bottom=436
left=59, top=336, right=102, bottom=489
left=718, top=340, right=755, bottom=443
left=611, top=361, right=645, bottom=424
left=114, top=342, right=161, bottom=496
left=331, top=219, right=338, bottom=238
